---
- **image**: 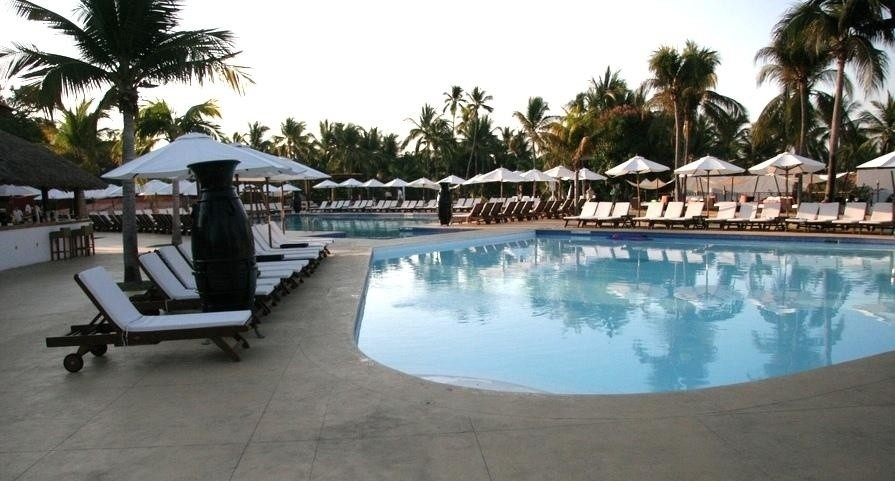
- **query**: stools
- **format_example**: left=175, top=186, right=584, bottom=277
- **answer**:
left=70, top=226, right=88, bottom=258
left=49, top=227, right=73, bottom=261
left=79, top=223, right=95, bottom=255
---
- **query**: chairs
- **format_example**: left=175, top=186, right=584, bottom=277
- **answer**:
left=46, top=266, right=254, bottom=372
left=87, top=194, right=335, bottom=338
left=301, top=195, right=596, bottom=226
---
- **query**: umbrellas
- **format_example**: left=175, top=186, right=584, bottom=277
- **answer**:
left=855, top=149, right=895, bottom=168
left=606, top=251, right=895, bottom=327
